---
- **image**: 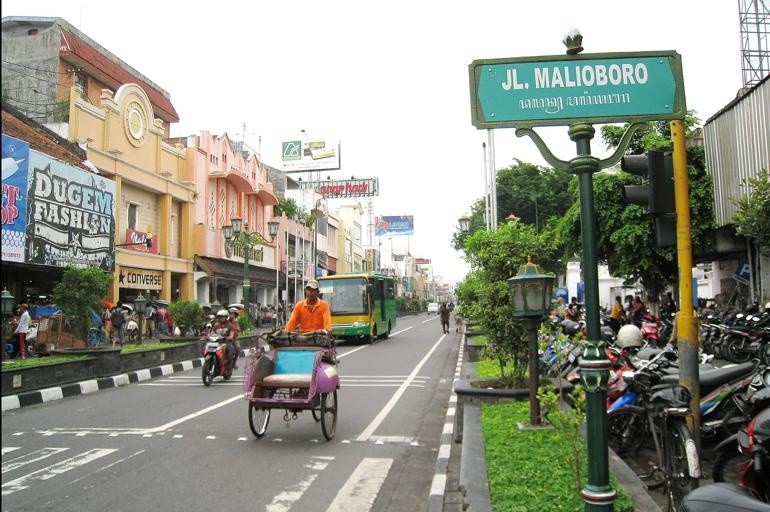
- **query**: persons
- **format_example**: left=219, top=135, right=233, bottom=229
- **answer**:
left=666, top=304, right=706, bottom=350
left=8, top=296, right=293, bottom=361
left=228, top=306, right=241, bottom=370
left=568, top=296, right=586, bottom=323
left=435, top=303, right=450, bottom=335
left=203, top=307, right=235, bottom=371
left=611, top=289, right=678, bottom=331
left=282, top=278, right=334, bottom=336
left=452, top=302, right=464, bottom=334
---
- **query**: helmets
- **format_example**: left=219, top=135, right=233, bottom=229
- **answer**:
left=618, top=325, right=643, bottom=349
left=228, top=308, right=239, bottom=313
left=217, top=310, right=228, bottom=317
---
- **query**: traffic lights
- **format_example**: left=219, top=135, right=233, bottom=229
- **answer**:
left=618, top=152, right=654, bottom=210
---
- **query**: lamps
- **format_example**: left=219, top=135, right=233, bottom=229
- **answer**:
left=115, top=237, right=153, bottom=250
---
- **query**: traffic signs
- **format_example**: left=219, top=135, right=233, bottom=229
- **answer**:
left=469, top=48, right=687, bottom=129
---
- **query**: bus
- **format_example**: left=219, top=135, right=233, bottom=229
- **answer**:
left=315, top=273, right=397, bottom=345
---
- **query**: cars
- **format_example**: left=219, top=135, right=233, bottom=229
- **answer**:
left=427, top=302, right=439, bottom=314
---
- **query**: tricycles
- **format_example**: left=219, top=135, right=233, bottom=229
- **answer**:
left=248, top=326, right=340, bottom=440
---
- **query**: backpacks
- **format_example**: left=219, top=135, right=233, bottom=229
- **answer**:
left=112, top=309, right=124, bottom=327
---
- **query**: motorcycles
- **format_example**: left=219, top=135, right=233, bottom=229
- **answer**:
left=201, top=337, right=236, bottom=386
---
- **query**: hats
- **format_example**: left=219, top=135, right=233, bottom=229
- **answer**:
left=19, top=304, right=29, bottom=310
left=305, top=280, right=319, bottom=289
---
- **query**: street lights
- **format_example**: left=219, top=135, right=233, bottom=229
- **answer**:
left=224, top=217, right=279, bottom=317
left=458, top=206, right=519, bottom=235
